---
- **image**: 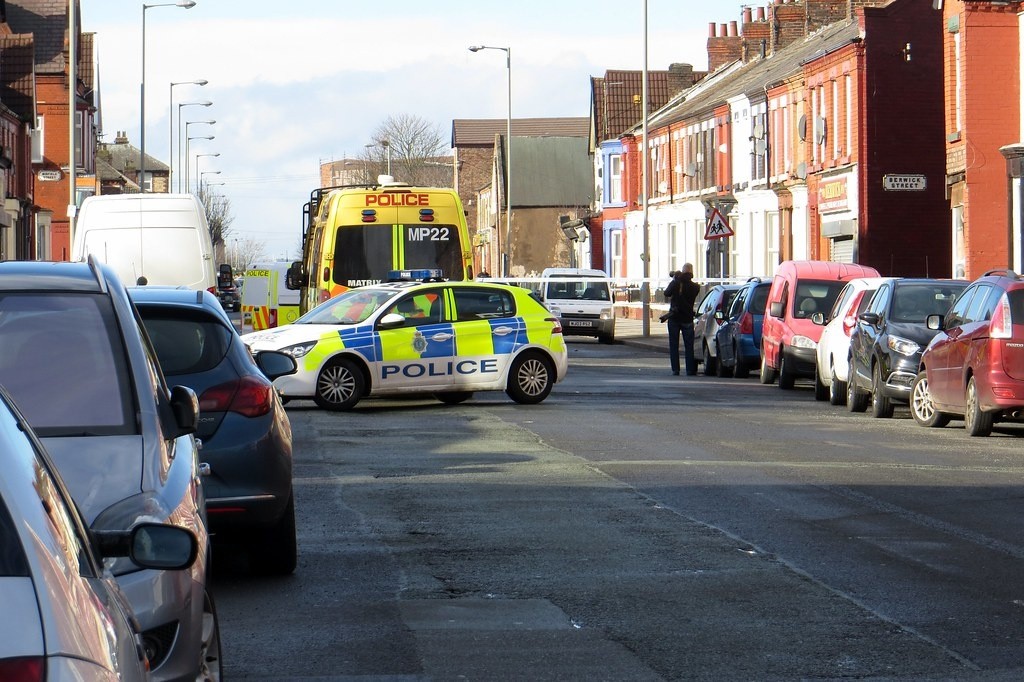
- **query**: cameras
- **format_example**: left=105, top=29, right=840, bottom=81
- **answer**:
left=670, top=270, right=681, bottom=277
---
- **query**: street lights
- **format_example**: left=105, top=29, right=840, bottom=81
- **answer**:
left=365, top=143, right=391, bottom=175
left=188, top=136, right=215, bottom=193
left=178, top=101, right=213, bottom=192
left=333, top=176, right=352, bottom=185
left=207, top=183, right=227, bottom=215
left=168, top=80, right=208, bottom=193
left=184, top=120, right=216, bottom=192
left=200, top=172, right=222, bottom=201
left=345, top=162, right=367, bottom=184
left=469, top=45, right=511, bottom=277
left=140, top=1, right=197, bottom=193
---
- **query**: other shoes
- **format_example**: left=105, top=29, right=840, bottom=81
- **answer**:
left=673, top=370, right=680, bottom=375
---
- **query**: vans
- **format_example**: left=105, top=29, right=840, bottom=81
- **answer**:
left=76, top=192, right=221, bottom=299
left=286, top=186, right=474, bottom=318
left=240, top=260, right=305, bottom=330
left=759, top=260, right=883, bottom=389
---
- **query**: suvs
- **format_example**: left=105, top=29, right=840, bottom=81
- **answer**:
left=714, top=276, right=773, bottom=379
left=909, top=268, right=1024, bottom=437
left=0, top=253, right=223, bottom=681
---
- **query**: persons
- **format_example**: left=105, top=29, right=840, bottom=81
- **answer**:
left=664, top=264, right=700, bottom=375
left=477, top=267, right=489, bottom=277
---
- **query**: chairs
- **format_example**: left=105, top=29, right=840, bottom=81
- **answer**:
left=11, top=329, right=119, bottom=426
left=555, top=283, right=570, bottom=297
left=430, top=299, right=440, bottom=318
left=582, top=288, right=596, bottom=298
left=896, top=295, right=926, bottom=320
left=397, top=298, right=416, bottom=322
left=796, top=298, right=818, bottom=318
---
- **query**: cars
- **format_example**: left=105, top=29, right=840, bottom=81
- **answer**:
left=692, top=285, right=744, bottom=376
left=813, top=278, right=908, bottom=404
left=127, top=286, right=297, bottom=575
left=216, top=272, right=244, bottom=313
left=243, top=268, right=570, bottom=414
left=846, top=277, right=973, bottom=418
left=0, top=389, right=200, bottom=681
left=539, top=268, right=616, bottom=343
